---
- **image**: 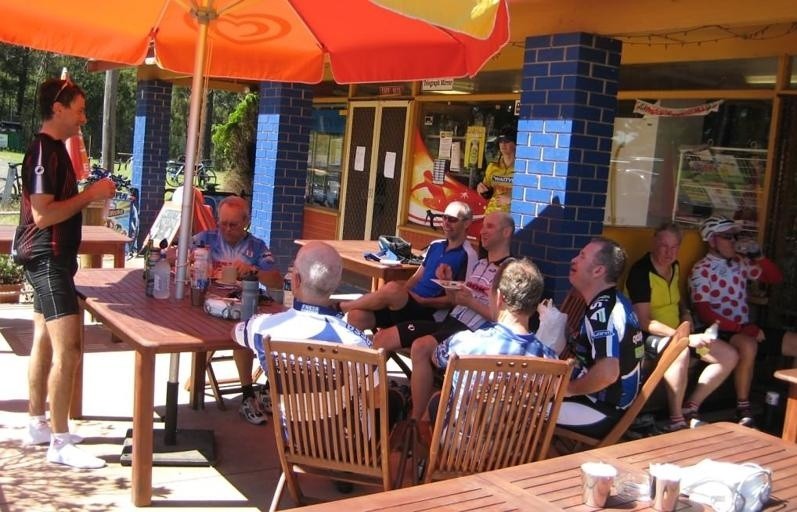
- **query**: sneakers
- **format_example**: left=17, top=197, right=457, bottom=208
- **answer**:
left=258, top=390, right=274, bottom=413
left=45, top=444, right=105, bottom=469
left=28, top=427, right=82, bottom=446
left=239, top=397, right=267, bottom=423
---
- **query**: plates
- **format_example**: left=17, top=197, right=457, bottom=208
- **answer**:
left=427, top=278, right=469, bottom=290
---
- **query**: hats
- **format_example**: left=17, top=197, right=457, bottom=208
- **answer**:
left=495, top=128, right=517, bottom=143
left=700, top=214, right=742, bottom=241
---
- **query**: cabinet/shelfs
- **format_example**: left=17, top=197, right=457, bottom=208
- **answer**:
left=672, top=146, right=769, bottom=229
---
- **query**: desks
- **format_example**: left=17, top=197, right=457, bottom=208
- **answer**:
left=69, top=267, right=291, bottom=506
left=294, top=240, right=425, bottom=292
left=773, top=368, right=797, bottom=443
left=277, top=420, right=796, bottom=512
left=0, top=225, right=132, bottom=344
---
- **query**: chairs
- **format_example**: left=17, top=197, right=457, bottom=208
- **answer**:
left=184, top=350, right=265, bottom=411
left=263, top=334, right=411, bottom=512
left=537, top=320, right=691, bottom=460
left=559, top=286, right=587, bottom=360
left=411, top=353, right=576, bottom=486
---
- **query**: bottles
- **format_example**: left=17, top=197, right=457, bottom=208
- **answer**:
left=143, top=238, right=259, bottom=322
left=694, top=318, right=719, bottom=356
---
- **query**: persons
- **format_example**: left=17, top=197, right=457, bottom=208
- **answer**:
left=417, top=256, right=563, bottom=480
left=621, top=220, right=740, bottom=434
left=328, top=199, right=479, bottom=337
left=475, top=129, right=516, bottom=218
left=369, top=210, right=520, bottom=461
left=13, top=78, right=117, bottom=471
left=162, top=194, right=288, bottom=426
left=540, top=237, right=644, bottom=440
left=684, top=214, right=797, bottom=430
left=410, top=169, right=463, bottom=212
left=229, top=240, right=412, bottom=494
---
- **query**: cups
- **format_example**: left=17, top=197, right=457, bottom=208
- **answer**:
left=648, top=471, right=681, bottom=512
left=578, top=462, right=618, bottom=509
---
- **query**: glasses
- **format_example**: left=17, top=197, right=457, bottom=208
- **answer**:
left=52, top=78, right=74, bottom=102
left=715, top=231, right=739, bottom=242
left=440, top=214, right=466, bottom=223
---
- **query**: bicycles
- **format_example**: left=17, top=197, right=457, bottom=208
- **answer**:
left=0, top=161, right=23, bottom=199
left=165, top=155, right=217, bottom=190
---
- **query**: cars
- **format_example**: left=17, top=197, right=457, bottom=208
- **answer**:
left=306, top=180, right=341, bottom=210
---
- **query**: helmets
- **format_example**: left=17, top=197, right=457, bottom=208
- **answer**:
left=380, top=235, right=410, bottom=257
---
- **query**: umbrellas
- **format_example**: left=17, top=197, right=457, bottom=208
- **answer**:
left=0, top=0, right=514, bottom=450
left=54, top=67, right=91, bottom=186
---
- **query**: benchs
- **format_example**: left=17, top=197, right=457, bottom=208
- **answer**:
left=602, top=225, right=708, bottom=387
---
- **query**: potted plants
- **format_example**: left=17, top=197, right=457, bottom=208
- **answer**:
left=0, top=254, right=24, bottom=303
